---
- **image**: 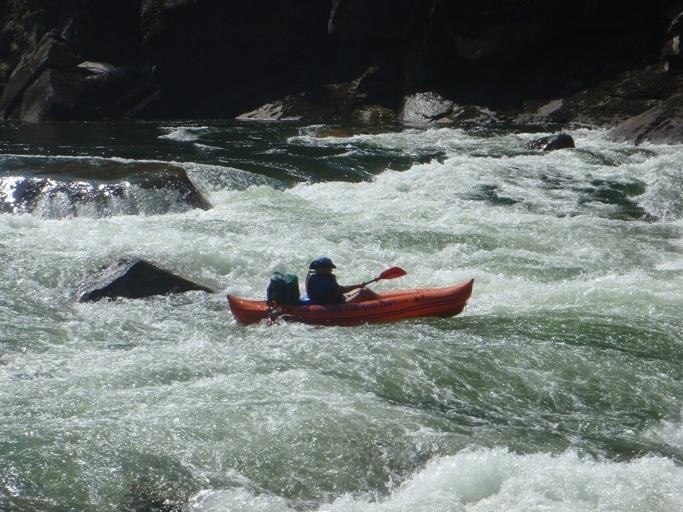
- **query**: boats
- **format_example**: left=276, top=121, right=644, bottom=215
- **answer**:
left=223, top=276, right=475, bottom=329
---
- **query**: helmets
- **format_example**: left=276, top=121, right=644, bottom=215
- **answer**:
left=315, top=257, right=336, bottom=268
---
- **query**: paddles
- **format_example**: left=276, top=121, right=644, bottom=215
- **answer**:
left=340, top=266, right=406, bottom=294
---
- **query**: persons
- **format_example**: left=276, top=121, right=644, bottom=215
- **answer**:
left=267, top=262, right=300, bottom=303
left=305, top=257, right=366, bottom=304
